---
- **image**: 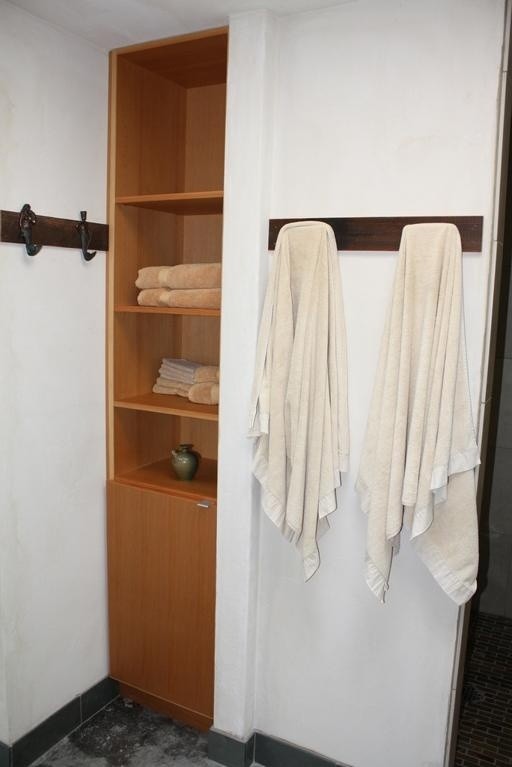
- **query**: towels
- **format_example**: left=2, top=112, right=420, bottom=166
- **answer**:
left=158, top=263, right=222, bottom=289
left=137, top=289, right=173, bottom=306
left=245, top=220, right=350, bottom=583
left=354, top=223, right=482, bottom=606
left=159, top=289, right=221, bottom=308
left=135, top=266, right=173, bottom=289
left=153, top=357, right=218, bottom=405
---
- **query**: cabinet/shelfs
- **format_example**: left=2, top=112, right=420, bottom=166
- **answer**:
left=107, top=25, right=229, bottom=732
